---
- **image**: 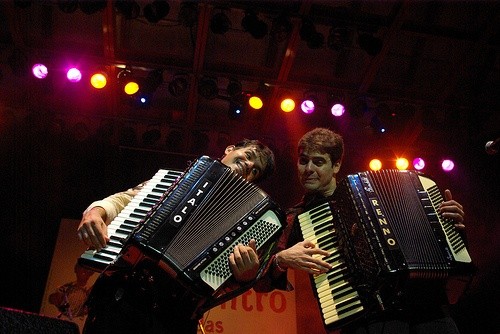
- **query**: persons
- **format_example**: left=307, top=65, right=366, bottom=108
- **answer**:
left=254, top=128, right=465, bottom=333
left=76, top=137, right=275, bottom=334
